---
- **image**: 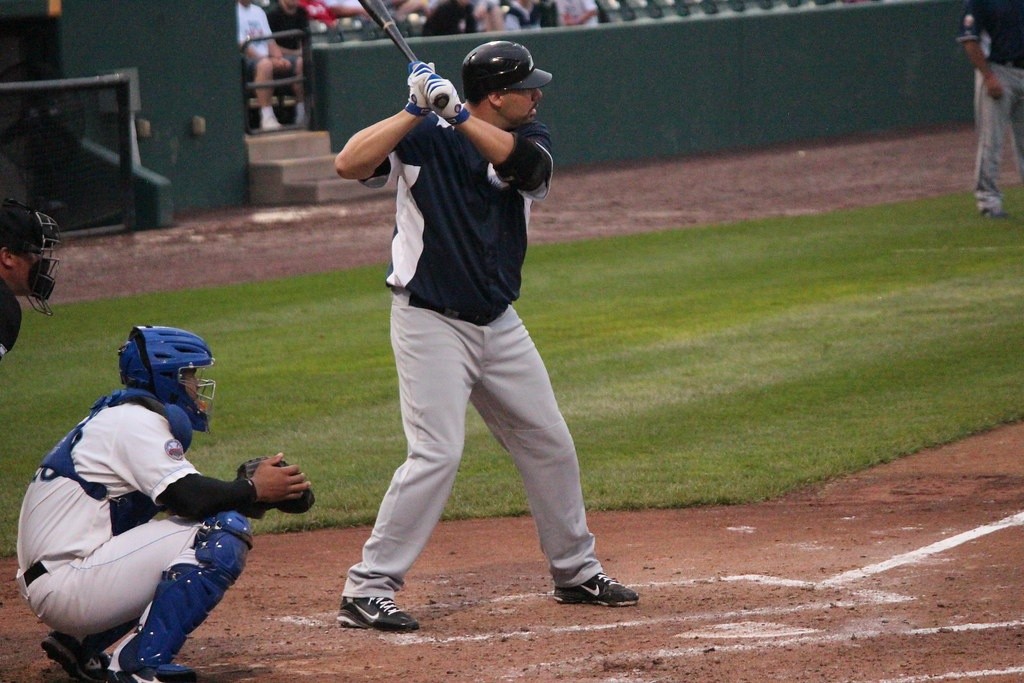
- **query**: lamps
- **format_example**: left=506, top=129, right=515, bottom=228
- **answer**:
left=136, top=119, right=151, bottom=141
left=193, top=115, right=207, bottom=138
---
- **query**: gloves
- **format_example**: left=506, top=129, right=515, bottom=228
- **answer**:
left=423, top=72, right=469, bottom=125
left=404, top=61, right=437, bottom=116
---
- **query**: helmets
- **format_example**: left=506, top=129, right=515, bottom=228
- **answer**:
left=120, top=325, right=216, bottom=433
left=461, top=40, right=553, bottom=103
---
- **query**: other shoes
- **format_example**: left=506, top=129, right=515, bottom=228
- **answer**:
left=295, top=103, right=307, bottom=125
left=259, top=105, right=285, bottom=131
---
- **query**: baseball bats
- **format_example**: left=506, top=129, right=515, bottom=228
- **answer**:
left=358, top=1, right=450, bottom=108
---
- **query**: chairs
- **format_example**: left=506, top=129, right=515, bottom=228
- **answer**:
left=327, top=18, right=377, bottom=45
left=364, top=16, right=409, bottom=40
left=406, top=0, right=838, bottom=35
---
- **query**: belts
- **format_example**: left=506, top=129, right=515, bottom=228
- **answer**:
left=991, top=57, right=1024, bottom=70
left=408, top=291, right=500, bottom=326
left=23, top=561, right=49, bottom=587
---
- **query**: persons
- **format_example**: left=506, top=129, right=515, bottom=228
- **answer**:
left=321, top=0, right=599, bottom=36
left=0, top=199, right=62, bottom=361
left=956, top=0, right=1024, bottom=218
left=235, top=0, right=341, bottom=130
left=334, top=41, right=639, bottom=632
left=17, top=325, right=312, bottom=683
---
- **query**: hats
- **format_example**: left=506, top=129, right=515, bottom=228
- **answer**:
left=0, top=200, right=58, bottom=253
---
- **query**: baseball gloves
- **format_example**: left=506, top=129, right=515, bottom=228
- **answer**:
left=235, top=455, right=316, bottom=521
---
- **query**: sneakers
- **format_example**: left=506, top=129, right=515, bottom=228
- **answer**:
left=336, top=596, right=419, bottom=632
left=552, top=573, right=638, bottom=607
left=109, top=668, right=164, bottom=683
left=41, top=630, right=115, bottom=683
